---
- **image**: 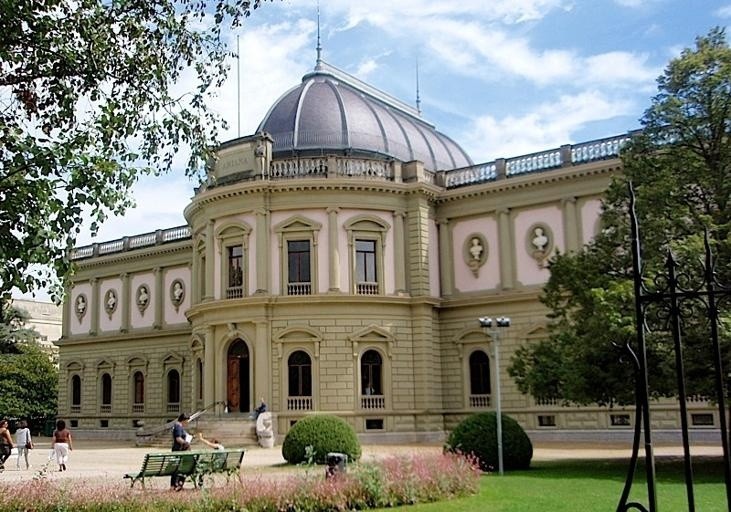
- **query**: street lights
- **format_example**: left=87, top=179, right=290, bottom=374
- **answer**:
left=479, top=315, right=512, bottom=476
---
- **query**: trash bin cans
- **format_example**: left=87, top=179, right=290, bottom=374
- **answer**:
left=44, top=420, right=55, bottom=437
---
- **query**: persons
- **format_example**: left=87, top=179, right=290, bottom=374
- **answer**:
left=235, top=266, right=243, bottom=298
left=77, top=297, right=85, bottom=312
left=531, top=227, right=548, bottom=250
left=0, top=420, right=16, bottom=473
left=198, top=432, right=228, bottom=487
left=50, top=420, right=73, bottom=472
left=229, top=265, right=236, bottom=298
left=14, top=420, right=34, bottom=471
left=173, top=283, right=183, bottom=299
left=469, top=238, right=484, bottom=259
left=169, top=412, right=192, bottom=492
left=138, top=288, right=148, bottom=304
left=108, top=292, right=115, bottom=308
left=365, top=383, right=375, bottom=395
left=254, top=398, right=268, bottom=420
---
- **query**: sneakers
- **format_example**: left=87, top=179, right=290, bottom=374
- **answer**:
left=0, top=464, right=66, bottom=472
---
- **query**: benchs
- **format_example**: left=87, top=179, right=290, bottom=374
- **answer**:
left=123, top=450, right=246, bottom=491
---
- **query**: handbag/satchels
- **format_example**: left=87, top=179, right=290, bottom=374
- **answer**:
left=25, top=430, right=34, bottom=450
